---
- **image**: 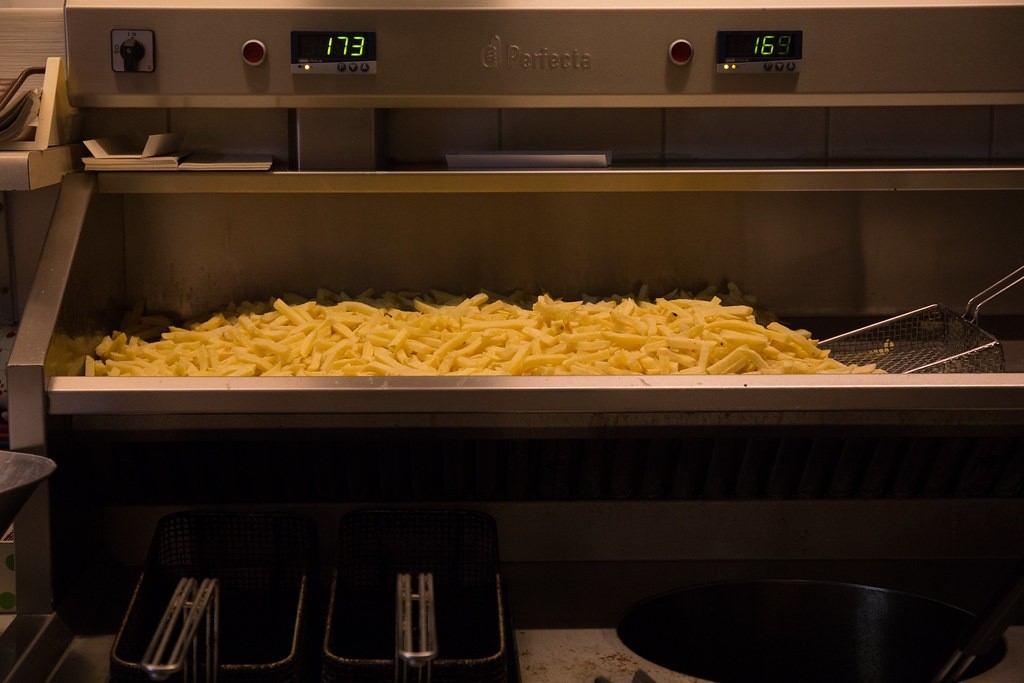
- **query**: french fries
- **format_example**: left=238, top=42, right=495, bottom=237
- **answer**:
left=84, top=292, right=889, bottom=374
